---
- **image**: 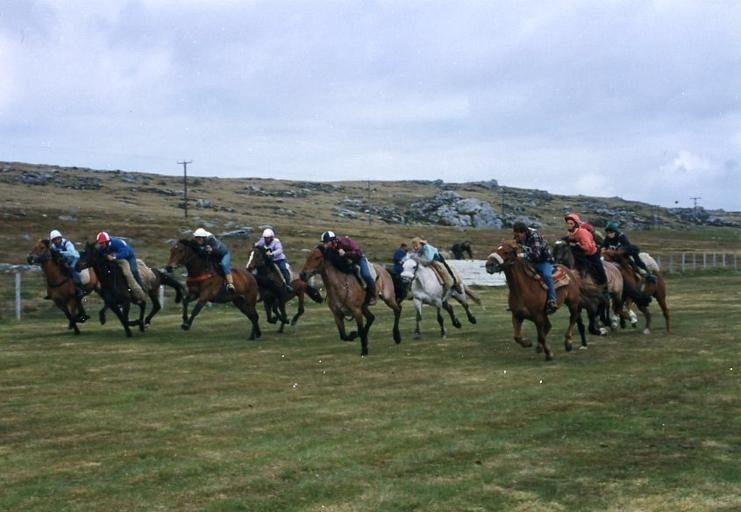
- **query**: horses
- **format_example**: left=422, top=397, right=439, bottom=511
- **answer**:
left=166, top=239, right=264, bottom=341
left=27, top=239, right=110, bottom=336
left=485, top=238, right=611, bottom=361
left=603, top=250, right=671, bottom=334
left=549, top=236, right=653, bottom=352
left=299, top=244, right=407, bottom=356
left=75, top=239, right=186, bottom=333
left=399, top=250, right=486, bottom=341
left=245, top=242, right=323, bottom=334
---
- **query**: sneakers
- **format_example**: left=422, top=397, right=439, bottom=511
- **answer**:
left=368, top=297, right=376, bottom=305
left=505, top=307, right=511, bottom=312
left=548, top=300, right=558, bottom=311
left=453, top=284, right=462, bottom=294
left=226, top=283, right=235, bottom=293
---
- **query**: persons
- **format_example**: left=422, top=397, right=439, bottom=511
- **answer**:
left=192, top=227, right=236, bottom=292
left=565, top=213, right=659, bottom=285
left=42, top=228, right=87, bottom=299
left=393, top=243, right=409, bottom=273
left=96, top=231, right=144, bottom=286
left=411, top=237, right=464, bottom=295
left=253, top=227, right=295, bottom=292
left=319, top=231, right=377, bottom=305
left=506, top=221, right=559, bottom=312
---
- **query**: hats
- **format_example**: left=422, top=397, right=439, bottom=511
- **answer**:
left=263, top=229, right=275, bottom=238
left=407, top=237, right=427, bottom=248
left=320, top=231, right=335, bottom=242
left=564, top=213, right=582, bottom=226
left=605, top=222, right=619, bottom=232
left=193, top=228, right=208, bottom=237
left=49, top=229, right=62, bottom=241
left=97, top=231, right=110, bottom=245
